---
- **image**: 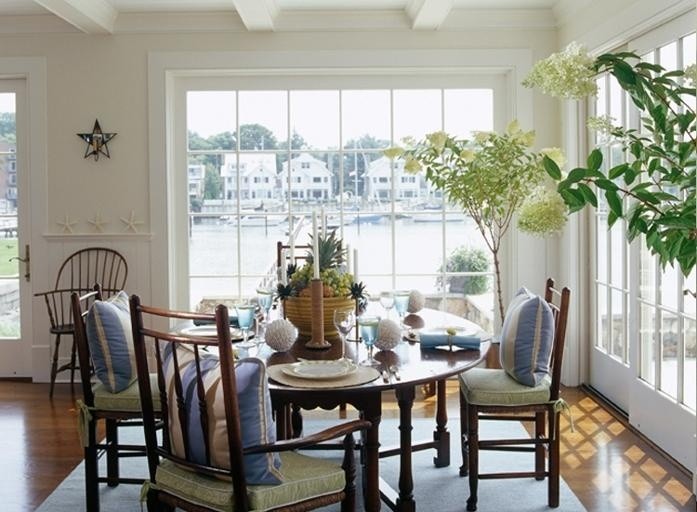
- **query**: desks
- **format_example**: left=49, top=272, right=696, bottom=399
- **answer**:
left=178, top=305, right=493, bottom=512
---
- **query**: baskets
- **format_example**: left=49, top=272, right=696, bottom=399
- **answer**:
left=279, top=296, right=360, bottom=338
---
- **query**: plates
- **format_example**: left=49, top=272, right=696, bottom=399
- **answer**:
left=180, top=326, right=235, bottom=337
left=281, top=360, right=359, bottom=379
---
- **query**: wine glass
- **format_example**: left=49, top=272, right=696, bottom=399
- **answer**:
left=333, top=310, right=381, bottom=366
left=379, top=288, right=413, bottom=330
left=235, top=288, right=273, bottom=347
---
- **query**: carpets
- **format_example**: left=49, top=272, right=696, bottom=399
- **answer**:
left=30, top=411, right=587, bottom=512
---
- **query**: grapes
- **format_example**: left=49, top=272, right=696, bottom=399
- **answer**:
left=290, top=264, right=353, bottom=295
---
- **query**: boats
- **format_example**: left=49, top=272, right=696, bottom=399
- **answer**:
left=218, top=210, right=466, bottom=233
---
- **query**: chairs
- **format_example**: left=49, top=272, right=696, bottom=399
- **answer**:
left=128, top=294, right=372, bottom=511
left=33, top=246, right=128, bottom=399
left=456, top=277, right=572, bottom=512
left=70, top=284, right=166, bottom=512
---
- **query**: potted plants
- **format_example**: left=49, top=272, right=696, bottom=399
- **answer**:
left=435, top=244, right=490, bottom=294
left=381, top=118, right=569, bottom=370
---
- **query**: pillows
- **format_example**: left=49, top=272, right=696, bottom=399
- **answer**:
left=496, top=286, right=557, bottom=387
left=163, top=340, right=286, bottom=484
left=85, top=291, right=140, bottom=395
